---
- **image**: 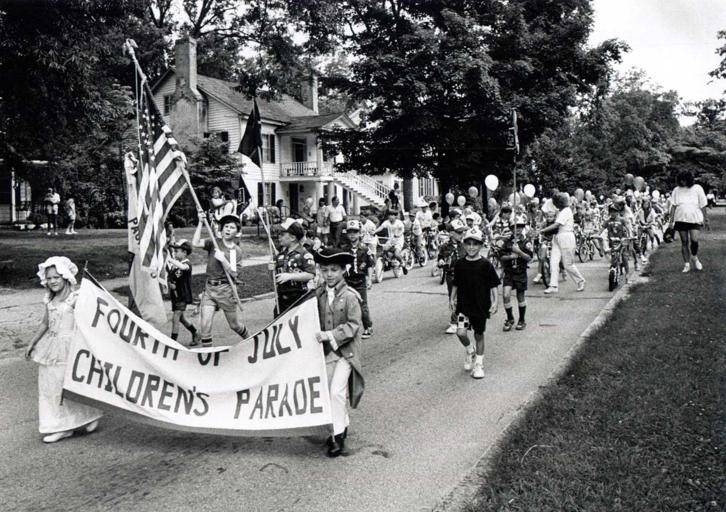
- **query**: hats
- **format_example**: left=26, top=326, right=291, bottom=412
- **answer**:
left=315, top=248, right=355, bottom=263
left=276, top=218, right=304, bottom=238
left=36, top=256, right=78, bottom=287
left=608, top=204, right=620, bottom=211
left=509, top=217, right=526, bottom=226
left=447, top=220, right=469, bottom=233
left=170, top=239, right=191, bottom=252
left=346, top=221, right=361, bottom=232
left=463, top=228, right=485, bottom=242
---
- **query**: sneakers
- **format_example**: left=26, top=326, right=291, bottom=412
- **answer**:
left=464, top=348, right=476, bottom=371
left=544, top=287, right=558, bottom=293
left=43, top=430, right=73, bottom=442
left=446, top=324, right=459, bottom=334
left=86, top=419, right=99, bottom=434
left=362, top=327, right=374, bottom=339
left=473, top=359, right=484, bottom=378
left=692, top=256, right=702, bottom=270
left=682, top=263, right=691, bottom=272
left=517, top=322, right=527, bottom=329
left=576, top=279, right=586, bottom=292
left=503, top=320, right=515, bottom=330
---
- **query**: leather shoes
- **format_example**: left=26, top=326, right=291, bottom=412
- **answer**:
left=328, top=434, right=341, bottom=457
left=189, top=331, right=201, bottom=346
left=327, top=427, right=347, bottom=443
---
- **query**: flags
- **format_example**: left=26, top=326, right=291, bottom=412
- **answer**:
left=136, top=77, right=189, bottom=293
left=238, top=98, right=263, bottom=168
left=504, top=110, right=519, bottom=156
left=123, top=151, right=168, bottom=329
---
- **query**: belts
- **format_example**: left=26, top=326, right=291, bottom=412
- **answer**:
left=206, top=280, right=228, bottom=285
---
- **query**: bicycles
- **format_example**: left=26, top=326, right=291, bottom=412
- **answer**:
left=400, top=223, right=437, bottom=270
left=487, top=226, right=512, bottom=280
left=575, top=231, right=595, bottom=263
left=606, top=220, right=654, bottom=292
left=431, top=229, right=450, bottom=285
left=541, top=234, right=552, bottom=288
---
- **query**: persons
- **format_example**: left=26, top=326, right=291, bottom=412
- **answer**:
left=339, top=222, right=373, bottom=339
left=44, top=183, right=60, bottom=235
left=651, top=198, right=670, bottom=234
left=584, top=202, right=613, bottom=224
left=631, top=200, right=655, bottom=251
left=573, top=205, right=588, bottom=254
left=415, top=204, right=433, bottom=235
left=276, top=200, right=284, bottom=216
left=64, top=196, right=78, bottom=235
left=438, top=229, right=467, bottom=334
left=24, top=256, right=103, bottom=443
left=304, top=248, right=363, bottom=458
left=450, top=229, right=500, bottom=379
left=402, top=212, right=425, bottom=262
left=389, top=182, right=400, bottom=210
left=210, top=186, right=232, bottom=222
left=165, top=238, right=202, bottom=346
left=582, top=213, right=595, bottom=256
left=373, top=210, right=409, bottom=277
left=591, top=202, right=629, bottom=277
left=488, top=206, right=513, bottom=253
left=303, top=195, right=314, bottom=216
left=441, top=205, right=497, bottom=230
left=301, top=206, right=316, bottom=239
left=380, top=199, right=390, bottom=223
left=625, top=195, right=636, bottom=215
left=528, top=202, right=544, bottom=253
left=208, top=192, right=234, bottom=222
left=514, top=204, right=529, bottom=239
left=538, top=210, right=560, bottom=241
left=164, top=215, right=175, bottom=258
left=322, top=196, right=347, bottom=249
left=317, top=199, right=329, bottom=228
left=431, top=213, right=442, bottom=231
left=669, top=170, right=711, bottom=274
left=498, top=216, right=533, bottom=331
left=429, top=202, right=436, bottom=216
left=357, top=212, right=378, bottom=267
left=706, top=189, right=717, bottom=207
left=536, top=192, right=587, bottom=294
left=268, top=216, right=316, bottom=320
left=201, top=216, right=255, bottom=347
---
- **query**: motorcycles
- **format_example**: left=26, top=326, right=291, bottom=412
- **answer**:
left=367, top=230, right=405, bottom=283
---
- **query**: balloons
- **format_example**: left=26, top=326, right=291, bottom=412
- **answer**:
left=627, top=188, right=633, bottom=196
left=457, top=195, right=466, bottom=207
left=468, top=186, right=478, bottom=198
left=524, top=184, right=535, bottom=197
left=633, top=186, right=671, bottom=200
left=625, top=173, right=634, bottom=185
left=445, top=193, right=454, bottom=205
left=585, top=190, right=591, bottom=200
left=574, top=188, right=584, bottom=203
left=600, top=194, right=604, bottom=200
left=509, top=191, right=520, bottom=206
left=606, top=189, right=626, bottom=203
left=489, top=197, right=497, bottom=207
left=633, top=176, right=644, bottom=188
left=485, top=174, right=499, bottom=192
